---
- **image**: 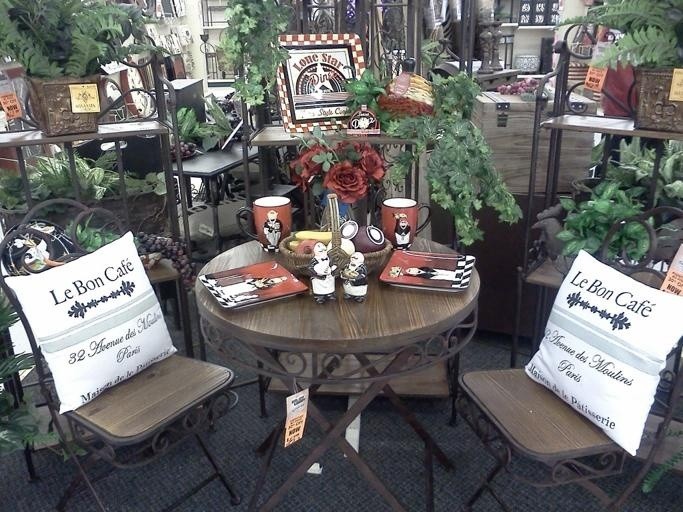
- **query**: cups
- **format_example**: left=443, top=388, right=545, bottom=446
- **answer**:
left=236, top=195, right=293, bottom=255
left=381, top=196, right=431, bottom=250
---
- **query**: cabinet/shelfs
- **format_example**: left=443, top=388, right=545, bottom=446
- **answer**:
left=0, top=1, right=208, bottom=483
left=169, top=1, right=476, bottom=431
left=508, top=24, right=683, bottom=373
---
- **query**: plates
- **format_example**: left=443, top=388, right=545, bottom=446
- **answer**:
left=378, top=250, right=477, bottom=291
left=198, top=259, right=308, bottom=312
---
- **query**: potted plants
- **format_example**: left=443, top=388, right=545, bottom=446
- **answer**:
left=0, top=2, right=167, bottom=137
left=558, top=1, right=683, bottom=134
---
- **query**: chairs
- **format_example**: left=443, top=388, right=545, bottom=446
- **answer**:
left=1, top=198, right=241, bottom=512
left=455, top=205, right=682, bottom=512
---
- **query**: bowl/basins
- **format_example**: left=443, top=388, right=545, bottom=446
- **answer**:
left=351, top=225, right=384, bottom=252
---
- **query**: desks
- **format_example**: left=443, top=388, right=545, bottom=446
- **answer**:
left=194, top=228, right=481, bottom=512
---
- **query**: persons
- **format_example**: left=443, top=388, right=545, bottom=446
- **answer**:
left=339, top=252, right=368, bottom=303
left=307, top=241, right=337, bottom=305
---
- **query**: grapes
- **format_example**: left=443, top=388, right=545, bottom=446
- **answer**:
left=217, top=99, right=233, bottom=113
left=136, top=230, right=197, bottom=290
left=169, top=141, right=198, bottom=158
left=497, top=77, right=539, bottom=96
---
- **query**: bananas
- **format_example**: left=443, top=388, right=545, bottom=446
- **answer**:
left=287, top=227, right=332, bottom=250
